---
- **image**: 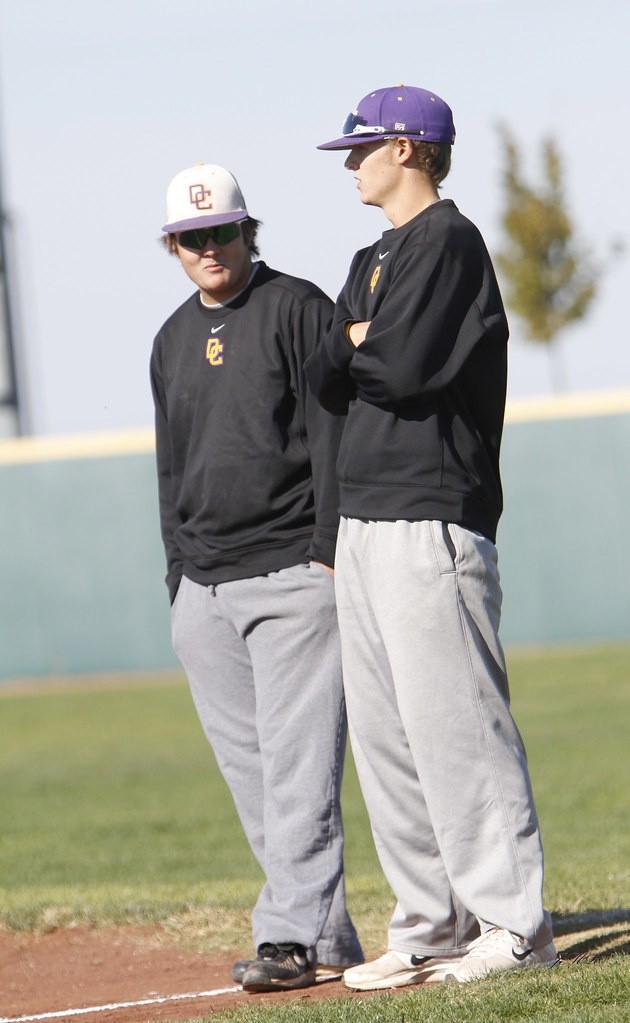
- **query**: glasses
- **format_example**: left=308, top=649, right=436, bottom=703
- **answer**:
left=173, top=218, right=249, bottom=249
left=342, top=112, right=425, bottom=137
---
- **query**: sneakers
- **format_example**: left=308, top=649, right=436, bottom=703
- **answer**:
left=342, top=949, right=464, bottom=991
left=242, top=942, right=318, bottom=990
left=233, top=957, right=365, bottom=983
left=445, top=928, right=558, bottom=982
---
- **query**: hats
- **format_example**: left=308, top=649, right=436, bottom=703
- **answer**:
left=161, top=163, right=247, bottom=233
left=317, top=84, right=456, bottom=150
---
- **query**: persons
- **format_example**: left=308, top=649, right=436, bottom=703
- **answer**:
left=148, top=163, right=365, bottom=993
left=302, top=84, right=559, bottom=989
